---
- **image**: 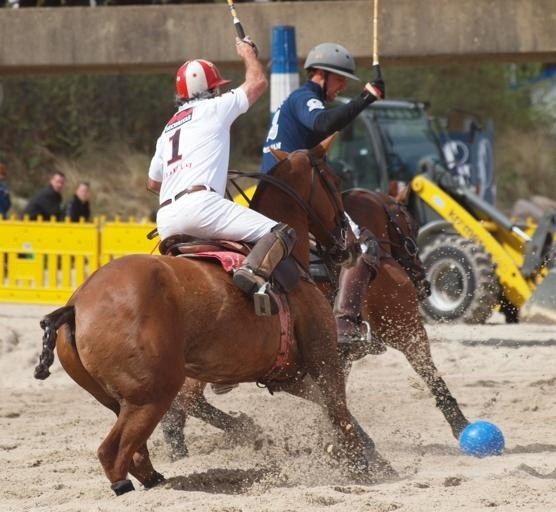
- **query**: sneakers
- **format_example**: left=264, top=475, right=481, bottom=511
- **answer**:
left=233, top=232, right=290, bottom=315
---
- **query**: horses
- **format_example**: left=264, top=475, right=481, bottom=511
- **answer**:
left=33, top=129, right=401, bottom=498
left=160, top=180, right=474, bottom=464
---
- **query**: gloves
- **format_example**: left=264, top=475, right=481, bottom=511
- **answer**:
left=359, top=79, right=386, bottom=105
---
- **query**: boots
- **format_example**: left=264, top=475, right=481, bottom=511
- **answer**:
left=333, top=253, right=388, bottom=355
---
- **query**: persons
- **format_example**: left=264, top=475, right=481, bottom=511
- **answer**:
left=19, top=167, right=67, bottom=220
left=58, top=180, right=94, bottom=223
left=146, top=33, right=298, bottom=318
left=260, top=37, right=396, bottom=354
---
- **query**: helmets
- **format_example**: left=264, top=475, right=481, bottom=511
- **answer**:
left=175, top=59, right=231, bottom=100
left=303, top=42, right=362, bottom=83
left=458, top=419, right=506, bottom=458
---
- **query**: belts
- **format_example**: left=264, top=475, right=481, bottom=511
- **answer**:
left=157, top=185, right=216, bottom=210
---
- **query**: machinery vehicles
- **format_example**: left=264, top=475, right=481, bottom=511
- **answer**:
left=234, top=94, right=556, bottom=323
